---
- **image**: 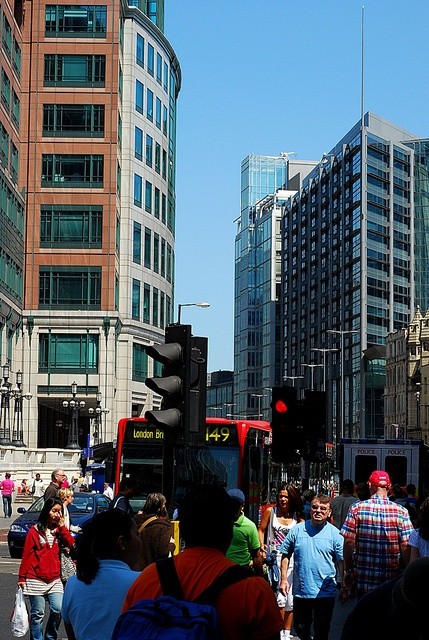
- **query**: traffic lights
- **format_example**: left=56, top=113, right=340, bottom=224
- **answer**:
left=303, top=391, right=327, bottom=462
left=270, top=385, right=298, bottom=463
left=145, top=325, right=191, bottom=446
left=189, top=337, right=207, bottom=433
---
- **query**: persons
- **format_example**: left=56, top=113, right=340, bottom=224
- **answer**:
left=19, top=478, right=29, bottom=493
left=222, top=488, right=264, bottom=570
left=331, top=478, right=358, bottom=527
left=408, top=496, right=429, bottom=562
left=405, top=483, right=418, bottom=503
left=394, top=485, right=407, bottom=506
left=100, top=482, right=113, bottom=499
left=63, top=510, right=143, bottom=638
left=0, top=474, right=14, bottom=521
left=129, top=491, right=174, bottom=569
left=342, top=470, right=416, bottom=602
left=18, top=495, right=75, bottom=640
left=278, top=495, right=344, bottom=639
left=39, top=470, right=67, bottom=527
left=120, top=480, right=284, bottom=638
left=28, top=473, right=45, bottom=492
left=303, top=490, right=315, bottom=518
left=257, top=484, right=303, bottom=637
left=340, top=555, right=428, bottom=639
left=53, top=487, right=76, bottom=509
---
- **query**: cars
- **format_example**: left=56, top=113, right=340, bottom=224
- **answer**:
left=7, top=492, right=111, bottom=558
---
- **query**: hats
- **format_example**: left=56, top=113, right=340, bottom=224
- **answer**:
left=227, top=488, right=246, bottom=503
left=367, top=470, right=391, bottom=487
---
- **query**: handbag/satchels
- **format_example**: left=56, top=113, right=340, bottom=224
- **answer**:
left=263, top=550, right=279, bottom=597
left=9, top=586, right=30, bottom=638
left=60, top=538, right=78, bottom=582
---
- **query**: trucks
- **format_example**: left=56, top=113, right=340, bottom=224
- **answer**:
left=340, top=439, right=429, bottom=499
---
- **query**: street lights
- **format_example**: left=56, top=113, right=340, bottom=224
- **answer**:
left=88, top=390, right=109, bottom=446
left=326, top=330, right=358, bottom=438
left=63, top=381, right=86, bottom=449
left=301, top=364, right=323, bottom=391
left=0, top=363, right=20, bottom=445
left=178, top=303, right=210, bottom=326
left=210, top=407, right=222, bottom=417
left=12, top=369, right=33, bottom=446
left=310, top=348, right=338, bottom=391
left=223, top=404, right=237, bottom=414
left=253, top=395, right=267, bottom=420
left=283, top=376, right=304, bottom=387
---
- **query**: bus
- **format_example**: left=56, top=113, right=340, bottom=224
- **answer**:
left=105, top=417, right=336, bottom=530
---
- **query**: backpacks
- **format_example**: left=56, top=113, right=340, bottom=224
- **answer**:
left=111, top=557, right=260, bottom=640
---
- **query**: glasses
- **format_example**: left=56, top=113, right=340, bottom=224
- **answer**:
left=312, top=506, right=331, bottom=512
left=56, top=474, right=65, bottom=478
